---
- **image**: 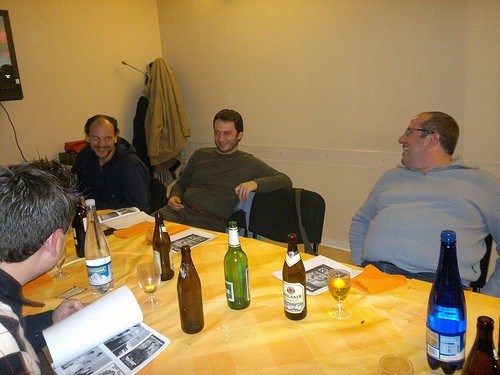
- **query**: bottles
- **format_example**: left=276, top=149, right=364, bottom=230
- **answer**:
left=177, top=245, right=204, bottom=334
left=224, top=221, right=251, bottom=310
left=84, top=199, right=115, bottom=294
left=460, top=316, right=499, bottom=375
left=426, top=230, right=466, bottom=375
left=282, top=234, right=308, bottom=320
left=152, top=211, right=174, bottom=281
left=71, top=198, right=87, bottom=258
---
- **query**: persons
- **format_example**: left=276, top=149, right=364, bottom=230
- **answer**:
left=0, top=158, right=82, bottom=375
left=105, top=329, right=138, bottom=356
left=349, top=111, right=500, bottom=298
left=71, top=115, right=150, bottom=213
left=63, top=351, right=102, bottom=375
left=122, top=342, right=155, bottom=369
left=149, top=109, right=292, bottom=234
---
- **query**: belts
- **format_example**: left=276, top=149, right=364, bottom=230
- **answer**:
left=182, top=200, right=225, bottom=222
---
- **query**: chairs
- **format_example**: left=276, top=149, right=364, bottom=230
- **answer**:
left=148, top=177, right=494, bottom=293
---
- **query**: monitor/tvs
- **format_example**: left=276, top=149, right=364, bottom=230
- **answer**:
left=0, top=9, right=23, bottom=101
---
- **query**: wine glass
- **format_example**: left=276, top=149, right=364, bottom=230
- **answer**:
left=328, top=269, right=352, bottom=319
left=52, top=246, right=71, bottom=281
left=137, top=262, right=161, bottom=310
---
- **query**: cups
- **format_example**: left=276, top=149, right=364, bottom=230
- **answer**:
left=378, top=354, right=415, bottom=375
left=147, top=219, right=157, bottom=244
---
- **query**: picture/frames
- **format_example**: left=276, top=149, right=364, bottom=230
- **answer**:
left=0, top=10, right=23, bottom=101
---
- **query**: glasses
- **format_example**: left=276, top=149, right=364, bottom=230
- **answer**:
left=404, top=127, right=437, bottom=137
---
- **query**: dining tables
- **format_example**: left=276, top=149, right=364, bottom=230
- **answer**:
left=18, top=209, right=500, bottom=375
